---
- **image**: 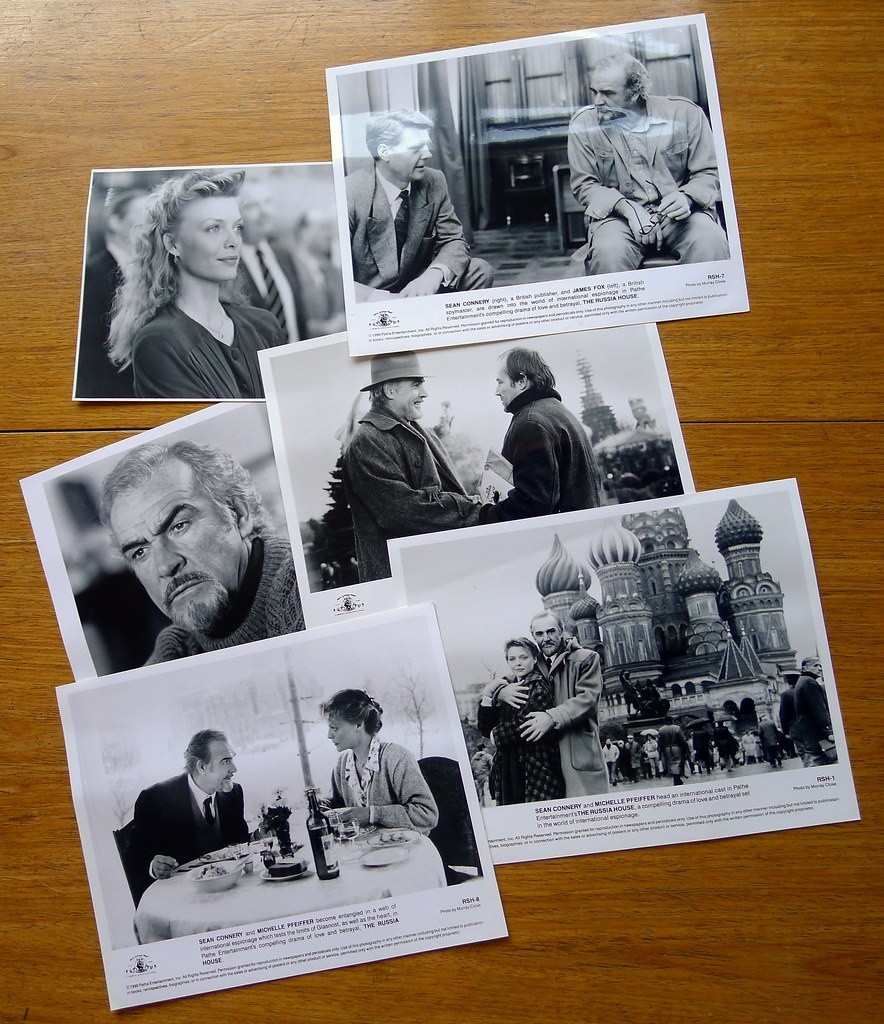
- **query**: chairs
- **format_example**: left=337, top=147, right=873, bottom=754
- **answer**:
left=112, top=816, right=154, bottom=905
left=416, top=758, right=485, bottom=886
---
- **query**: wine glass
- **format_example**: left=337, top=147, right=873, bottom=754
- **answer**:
left=340, top=818, right=359, bottom=852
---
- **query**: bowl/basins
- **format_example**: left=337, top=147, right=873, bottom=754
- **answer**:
left=186, top=861, right=244, bottom=893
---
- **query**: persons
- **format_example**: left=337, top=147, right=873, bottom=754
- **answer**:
left=76, top=443, right=306, bottom=672
left=76, top=170, right=345, bottom=399
left=134, top=728, right=270, bottom=903
left=345, top=111, right=495, bottom=304
left=320, top=347, right=683, bottom=591
left=308, top=687, right=455, bottom=888
left=469, top=613, right=835, bottom=807
left=568, top=54, right=731, bottom=275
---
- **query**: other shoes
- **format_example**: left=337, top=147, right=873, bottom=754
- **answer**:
left=612, top=753, right=798, bottom=788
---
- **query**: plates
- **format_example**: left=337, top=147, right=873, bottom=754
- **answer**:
left=365, top=829, right=422, bottom=847
left=324, top=807, right=356, bottom=820
left=199, top=848, right=244, bottom=863
left=359, top=846, right=410, bottom=867
left=260, top=869, right=305, bottom=881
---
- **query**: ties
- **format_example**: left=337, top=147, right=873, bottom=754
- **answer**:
left=203, top=795, right=215, bottom=826
left=393, top=189, right=410, bottom=266
left=547, top=658, right=552, bottom=665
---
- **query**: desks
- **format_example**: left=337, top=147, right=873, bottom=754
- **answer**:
left=136, top=828, right=447, bottom=945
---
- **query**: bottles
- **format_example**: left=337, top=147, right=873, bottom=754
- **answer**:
left=244, top=861, right=254, bottom=874
left=306, top=789, right=340, bottom=880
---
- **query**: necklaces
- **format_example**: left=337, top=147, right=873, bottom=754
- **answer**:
left=180, top=302, right=225, bottom=339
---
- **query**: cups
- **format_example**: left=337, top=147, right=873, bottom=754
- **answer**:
left=228, top=843, right=242, bottom=860
left=258, top=825, right=274, bottom=851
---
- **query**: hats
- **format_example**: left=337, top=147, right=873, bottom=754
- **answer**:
left=783, top=671, right=801, bottom=681
left=359, top=350, right=433, bottom=394
left=606, top=739, right=611, bottom=745
left=477, top=743, right=486, bottom=750
left=627, top=735, right=633, bottom=738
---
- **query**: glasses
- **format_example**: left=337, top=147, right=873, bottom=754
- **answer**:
left=624, top=179, right=666, bottom=235
